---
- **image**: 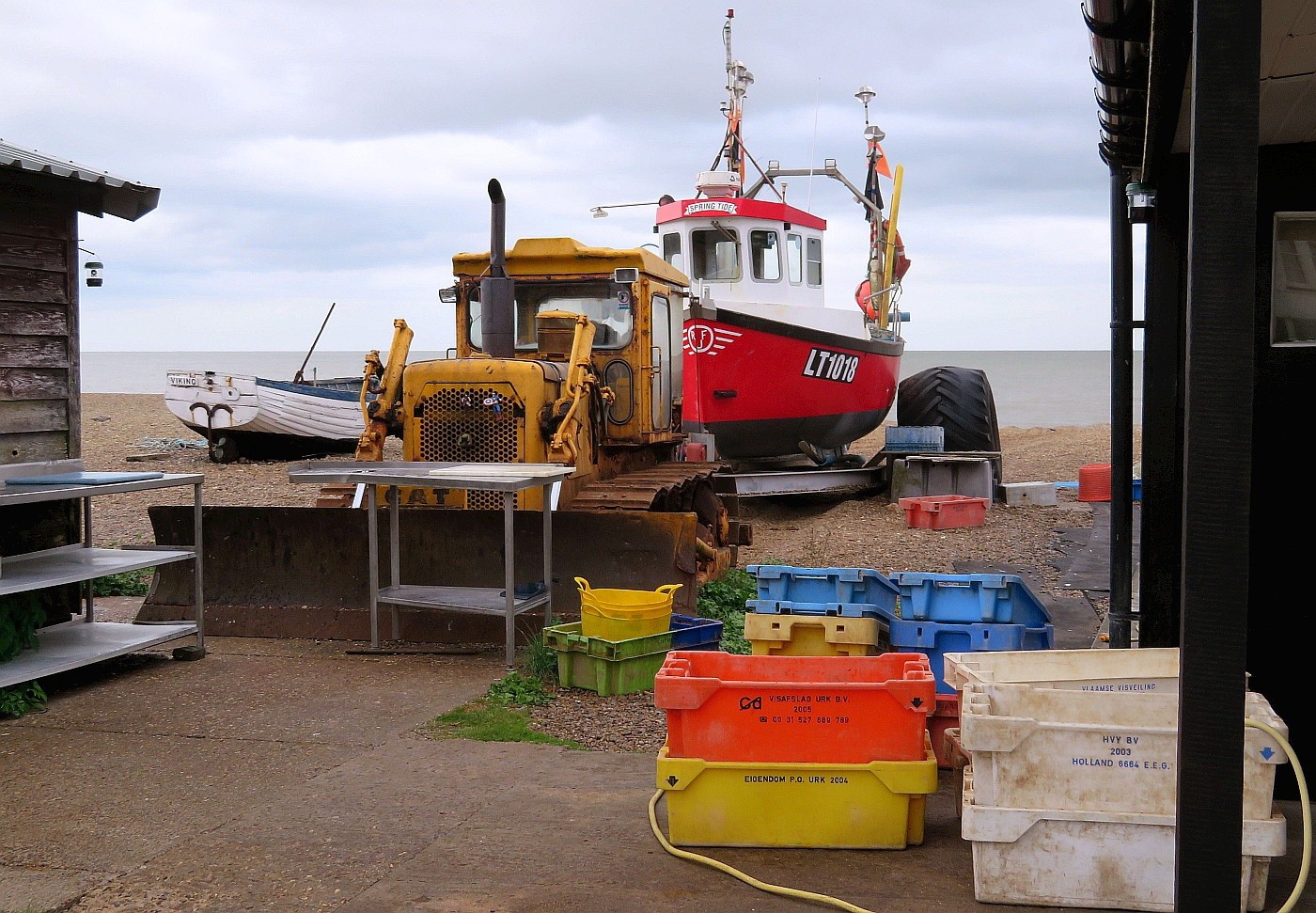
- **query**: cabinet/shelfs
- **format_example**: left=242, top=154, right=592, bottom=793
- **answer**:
left=1, top=470, right=206, bottom=696
left=369, top=481, right=550, bottom=670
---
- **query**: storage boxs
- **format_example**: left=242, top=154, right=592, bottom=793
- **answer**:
left=890, top=618, right=1054, bottom=694
left=888, top=570, right=1052, bottom=629
left=746, top=561, right=901, bottom=612
left=657, top=732, right=937, bottom=850
left=544, top=613, right=725, bottom=697
left=746, top=598, right=900, bottom=625
left=963, top=681, right=1288, bottom=819
left=898, top=494, right=990, bottom=529
left=887, top=426, right=945, bottom=452
left=744, top=613, right=897, bottom=657
left=947, top=727, right=973, bottom=816
left=942, top=647, right=1251, bottom=693
left=654, top=649, right=935, bottom=763
left=928, top=694, right=966, bottom=769
left=960, top=764, right=1287, bottom=912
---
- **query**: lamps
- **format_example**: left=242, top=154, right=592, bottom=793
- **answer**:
left=78, top=248, right=103, bottom=287
left=1124, top=181, right=1157, bottom=223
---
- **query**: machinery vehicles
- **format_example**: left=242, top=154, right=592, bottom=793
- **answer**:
left=128, top=178, right=755, bottom=648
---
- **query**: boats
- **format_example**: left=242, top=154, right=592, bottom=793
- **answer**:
left=163, top=303, right=379, bottom=461
left=584, top=4, right=912, bottom=467
